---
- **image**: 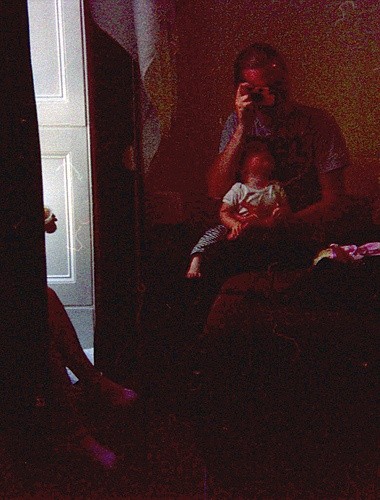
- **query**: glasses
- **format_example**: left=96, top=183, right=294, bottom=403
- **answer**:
left=244, top=75, right=290, bottom=103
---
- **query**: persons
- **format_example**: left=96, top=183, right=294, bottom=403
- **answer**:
left=205, top=40, right=352, bottom=228
left=189, top=137, right=292, bottom=278
left=42, top=208, right=138, bottom=472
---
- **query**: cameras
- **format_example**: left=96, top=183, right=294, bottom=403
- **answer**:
left=242, top=84, right=278, bottom=110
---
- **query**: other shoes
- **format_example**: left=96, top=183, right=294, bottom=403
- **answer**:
left=72, top=432, right=118, bottom=477
left=79, top=372, right=137, bottom=412
